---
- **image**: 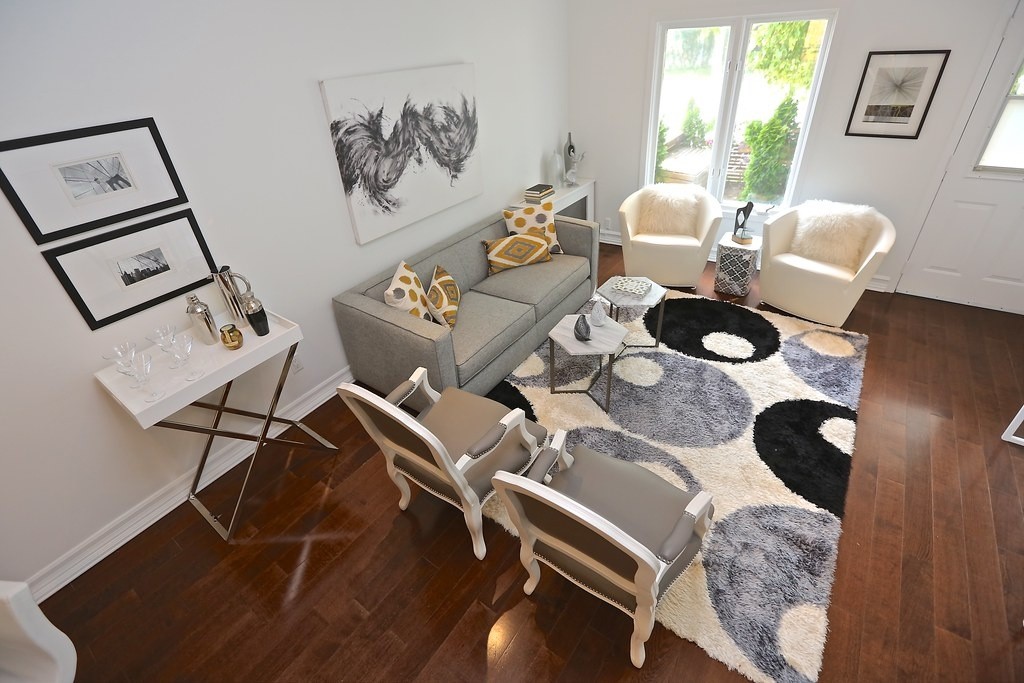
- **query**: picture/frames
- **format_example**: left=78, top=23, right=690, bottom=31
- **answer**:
left=845, top=50, right=952, bottom=139
left=0, top=117, right=189, bottom=245
left=41, top=208, right=219, bottom=331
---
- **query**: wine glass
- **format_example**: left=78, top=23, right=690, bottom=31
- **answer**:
left=101, top=341, right=150, bottom=388
left=145, top=325, right=190, bottom=369
left=160, top=334, right=204, bottom=381
left=115, top=351, right=166, bottom=403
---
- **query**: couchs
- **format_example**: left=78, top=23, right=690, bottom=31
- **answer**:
left=619, top=182, right=723, bottom=290
left=760, top=200, right=896, bottom=327
left=332, top=208, right=600, bottom=412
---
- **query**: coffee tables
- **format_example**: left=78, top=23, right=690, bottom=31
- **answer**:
left=548, top=276, right=668, bottom=413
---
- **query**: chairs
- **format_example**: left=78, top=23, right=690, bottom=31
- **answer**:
left=336, top=367, right=549, bottom=560
left=491, top=430, right=714, bottom=669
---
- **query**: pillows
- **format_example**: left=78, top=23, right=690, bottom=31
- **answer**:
left=501, top=202, right=564, bottom=253
left=481, top=226, right=554, bottom=276
left=384, top=260, right=434, bottom=322
left=424, top=265, right=463, bottom=332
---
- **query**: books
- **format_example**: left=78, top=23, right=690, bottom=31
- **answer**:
left=525, top=184, right=556, bottom=204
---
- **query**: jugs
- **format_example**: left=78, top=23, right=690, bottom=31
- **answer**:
left=207, top=265, right=252, bottom=330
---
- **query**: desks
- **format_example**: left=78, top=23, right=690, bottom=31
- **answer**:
left=510, top=179, right=596, bottom=221
left=94, top=308, right=340, bottom=543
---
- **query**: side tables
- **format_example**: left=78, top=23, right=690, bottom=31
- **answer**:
left=714, top=232, right=763, bottom=297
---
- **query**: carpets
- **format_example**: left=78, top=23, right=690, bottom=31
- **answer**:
left=481, top=286, right=869, bottom=683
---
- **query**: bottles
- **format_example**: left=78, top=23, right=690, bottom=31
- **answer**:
left=564, top=131, right=576, bottom=173
left=220, top=324, right=244, bottom=350
left=243, top=291, right=270, bottom=336
left=186, top=294, right=220, bottom=346
